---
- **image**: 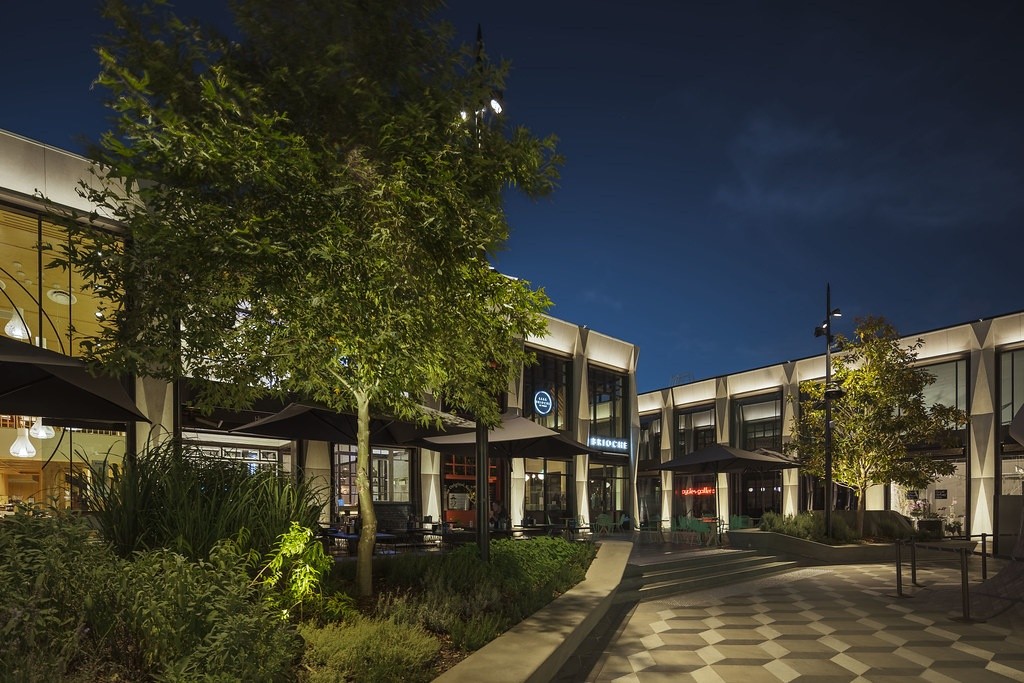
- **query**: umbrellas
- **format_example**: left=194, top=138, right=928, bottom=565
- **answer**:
left=420, top=410, right=601, bottom=540
left=644, top=443, right=792, bottom=549
left=749, top=448, right=807, bottom=516
left=228, top=392, right=477, bottom=556
left=0, top=334, right=153, bottom=425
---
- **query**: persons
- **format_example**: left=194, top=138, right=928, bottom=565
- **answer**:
left=489, top=501, right=509, bottom=529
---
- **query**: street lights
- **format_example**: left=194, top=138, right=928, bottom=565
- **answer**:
left=814, top=282, right=846, bottom=539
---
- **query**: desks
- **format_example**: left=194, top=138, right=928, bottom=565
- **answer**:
left=321, top=503, right=765, bottom=556
